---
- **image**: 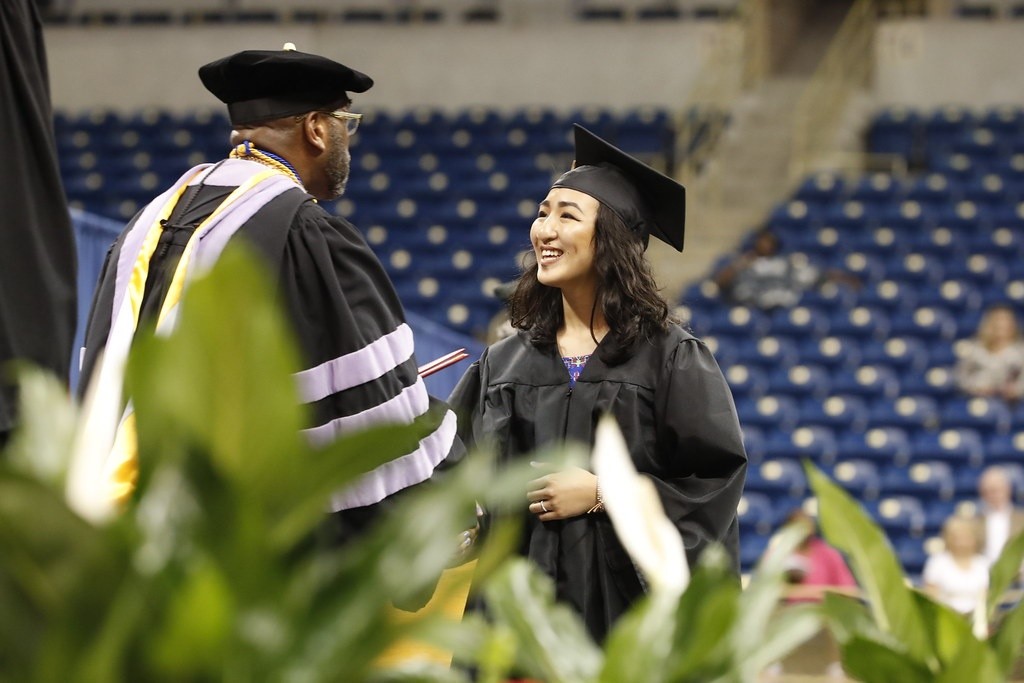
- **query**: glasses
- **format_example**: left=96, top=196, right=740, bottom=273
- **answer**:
left=291, top=109, right=361, bottom=135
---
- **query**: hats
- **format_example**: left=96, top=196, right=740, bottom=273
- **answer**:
left=198, top=42, right=372, bottom=124
left=551, top=121, right=687, bottom=254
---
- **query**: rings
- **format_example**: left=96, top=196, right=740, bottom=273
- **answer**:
left=541, top=501, right=547, bottom=512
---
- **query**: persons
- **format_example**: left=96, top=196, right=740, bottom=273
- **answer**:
left=921, top=464, right=1024, bottom=642
left=0, top=0, right=79, bottom=455
left=444, top=123, right=748, bottom=650
left=781, top=516, right=857, bottom=605
left=946, top=301, right=1024, bottom=413
left=63, top=41, right=466, bottom=628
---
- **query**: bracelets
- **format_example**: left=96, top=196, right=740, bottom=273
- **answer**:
left=587, top=477, right=604, bottom=514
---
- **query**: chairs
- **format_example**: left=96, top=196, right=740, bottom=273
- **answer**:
left=60, top=104, right=1023, bottom=576
